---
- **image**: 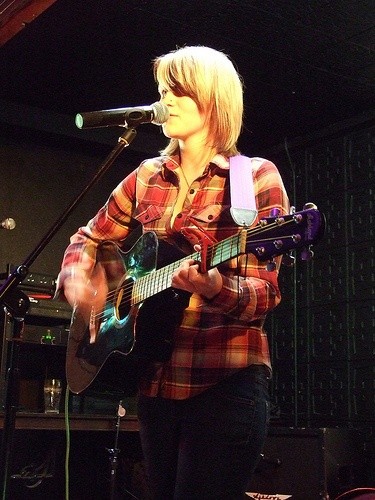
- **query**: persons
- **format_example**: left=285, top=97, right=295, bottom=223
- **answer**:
left=54, top=42, right=291, bottom=500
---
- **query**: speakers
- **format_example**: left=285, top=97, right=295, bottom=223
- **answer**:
left=0, top=413, right=147, bottom=500
left=243, top=427, right=327, bottom=499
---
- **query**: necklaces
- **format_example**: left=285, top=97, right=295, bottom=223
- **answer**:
left=179, top=163, right=190, bottom=190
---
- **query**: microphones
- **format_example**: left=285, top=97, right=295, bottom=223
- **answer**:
left=75, top=101, right=170, bottom=129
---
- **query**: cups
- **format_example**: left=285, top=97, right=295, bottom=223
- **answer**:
left=44, top=380, right=62, bottom=414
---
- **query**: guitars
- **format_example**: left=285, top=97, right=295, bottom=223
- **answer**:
left=67, top=203, right=328, bottom=397
